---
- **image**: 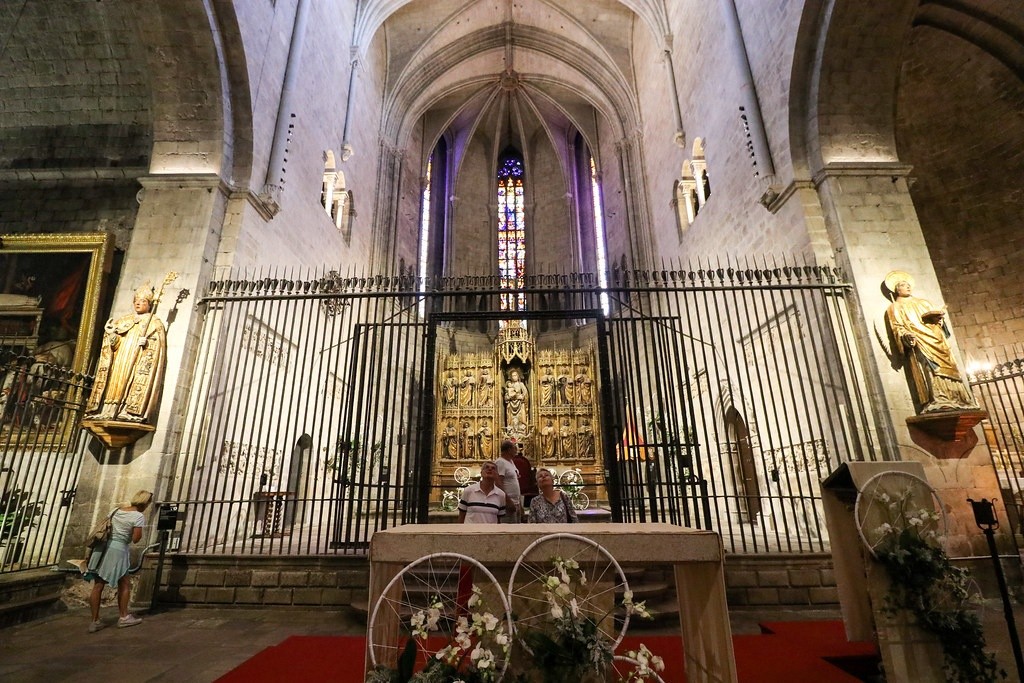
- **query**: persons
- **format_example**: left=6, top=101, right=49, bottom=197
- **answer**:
left=478, top=369, right=493, bottom=406
left=504, top=369, right=529, bottom=430
left=575, top=368, right=591, bottom=404
left=888, top=279, right=981, bottom=414
left=541, top=420, right=557, bottom=457
left=443, top=420, right=457, bottom=459
left=478, top=420, right=493, bottom=459
left=88, top=490, right=153, bottom=632
left=577, top=419, right=594, bottom=457
left=460, top=422, right=474, bottom=458
left=528, top=469, right=579, bottom=524
left=458, top=462, right=507, bottom=525
left=443, top=372, right=458, bottom=405
left=540, top=367, right=555, bottom=405
left=560, top=419, right=576, bottom=458
left=461, top=370, right=475, bottom=405
left=557, top=367, right=574, bottom=404
left=86, top=281, right=166, bottom=423
left=494, top=440, right=522, bottom=524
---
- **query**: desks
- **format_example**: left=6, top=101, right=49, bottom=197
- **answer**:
left=364, top=522, right=738, bottom=683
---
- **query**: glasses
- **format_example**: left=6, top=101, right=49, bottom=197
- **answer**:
left=515, top=470, right=519, bottom=477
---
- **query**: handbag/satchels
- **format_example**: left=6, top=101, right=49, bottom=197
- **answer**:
left=86, top=507, right=120, bottom=550
left=87, top=544, right=103, bottom=570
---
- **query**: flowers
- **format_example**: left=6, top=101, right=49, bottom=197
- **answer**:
left=399, top=559, right=666, bottom=683
left=879, top=490, right=1009, bottom=683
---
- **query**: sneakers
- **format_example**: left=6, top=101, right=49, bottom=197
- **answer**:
left=117, top=614, right=142, bottom=627
left=89, top=618, right=109, bottom=632
